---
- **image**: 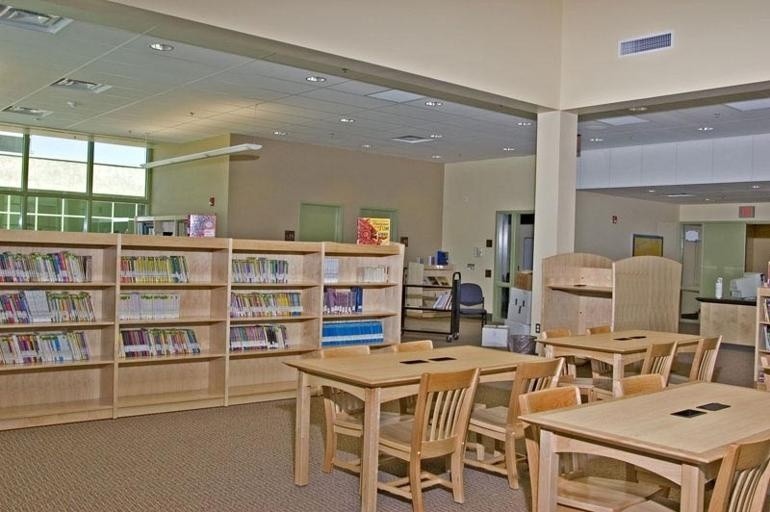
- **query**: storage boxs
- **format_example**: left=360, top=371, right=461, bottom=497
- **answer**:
left=515, top=271, right=531, bottom=290
left=504, top=321, right=531, bottom=336
left=483, top=325, right=511, bottom=347
left=508, top=289, right=532, bottom=323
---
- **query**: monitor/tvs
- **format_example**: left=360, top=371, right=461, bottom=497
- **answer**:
left=729, top=272, right=765, bottom=301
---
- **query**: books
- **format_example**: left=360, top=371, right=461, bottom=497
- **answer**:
left=356, top=216, right=390, bottom=245
left=232, top=256, right=290, bottom=282
left=0, top=331, right=89, bottom=363
left=405, top=287, right=423, bottom=307
left=0, top=251, right=92, bottom=283
left=121, top=253, right=191, bottom=283
left=120, top=329, right=201, bottom=358
left=188, top=214, right=216, bottom=236
left=322, top=321, right=384, bottom=347
left=119, top=294, right=180, bottom=320
left=230, top=324, right=288, bottom=353
left=432, top=293, right=452, bottom=310
left=230, top=292, right=302, bottom=318
left=323, top=287, right=362, bottom=315
left=0, top=288, right=96, bottom=323
left=356, top=264, right=390, bottom=282
left=324, top=258, right=339, bottom=284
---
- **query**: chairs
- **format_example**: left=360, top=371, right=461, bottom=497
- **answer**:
left=623, top=440, right=769, bottom=512
left=452, top=284, right=487, bottom=329
left=399, top=395, right=486, bottom=471
left=521, top=386, right=670, bottom=512
left=618, top=375, right=668, bottom=481
left=669, top=336, right=723, bottom=383
left=324, top=387, right=417, bottom=475
left=589, top=341, right=678, bottom=400
left=463, top=358, right=564, bottom=490
left=542, top=329, right=575, bottom=382
left=359, top=367, right=481, bottom=511
left=588, top=327, right=637, bottom=379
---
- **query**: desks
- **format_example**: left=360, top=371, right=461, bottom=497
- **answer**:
left=517, top=382, right=770, bottom=511
left=538, top=329, right=717, bottom=397
left=280, top=345, right=544, bottom=487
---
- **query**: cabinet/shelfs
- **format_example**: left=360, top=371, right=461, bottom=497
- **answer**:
left=756, top=288, right=770, bottom=382
left=0, top=229, right=405, bottom=429
left=410, top=261, right=454, bottom=319
left=541, top=253, right=681, bottom=339
left=135, top=213, right=191, bottom=236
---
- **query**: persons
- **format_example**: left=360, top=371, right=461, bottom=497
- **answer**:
left=137, top=222, right=155, bottom=236
